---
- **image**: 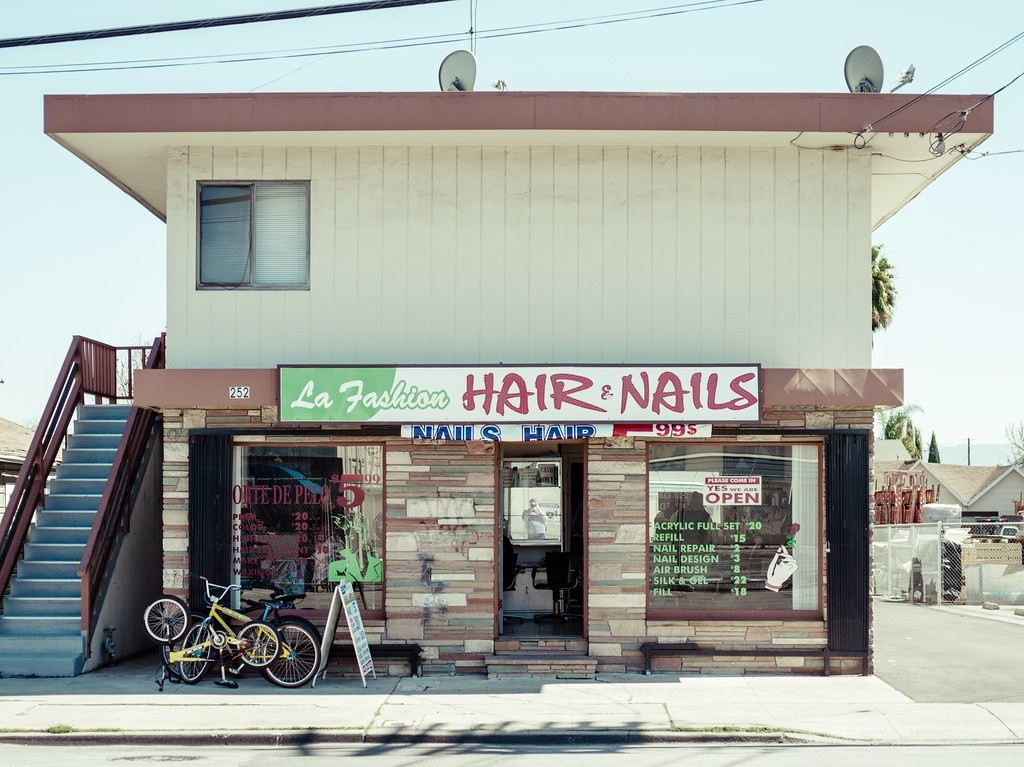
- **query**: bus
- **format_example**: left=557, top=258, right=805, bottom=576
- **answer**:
left=536, top=503, right=560, bottom=515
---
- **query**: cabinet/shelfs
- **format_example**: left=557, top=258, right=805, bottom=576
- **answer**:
left=502, top=542, right=564, bottom=619
left=502, top=456, right=562, bottom=542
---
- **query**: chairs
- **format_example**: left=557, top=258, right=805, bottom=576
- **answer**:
left=529, top=551, right=581, bottom=622
left=500, top=552, right=524, bottom=626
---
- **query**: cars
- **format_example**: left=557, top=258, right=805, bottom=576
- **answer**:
left=998, top=515, right=1022, bottom=521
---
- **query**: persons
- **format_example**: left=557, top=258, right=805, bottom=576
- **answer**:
left=522, top=499, right=547, bottom=539
left=654, top=492, right=718, bottom=545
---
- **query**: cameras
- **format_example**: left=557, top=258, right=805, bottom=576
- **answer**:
left=531, top=504, right=536, bottom=507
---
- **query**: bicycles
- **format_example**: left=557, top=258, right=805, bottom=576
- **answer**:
left=139, top=570, right=322, bottom=691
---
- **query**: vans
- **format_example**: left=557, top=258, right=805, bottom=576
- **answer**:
left=994, top=526, right=1019, bottom=537
